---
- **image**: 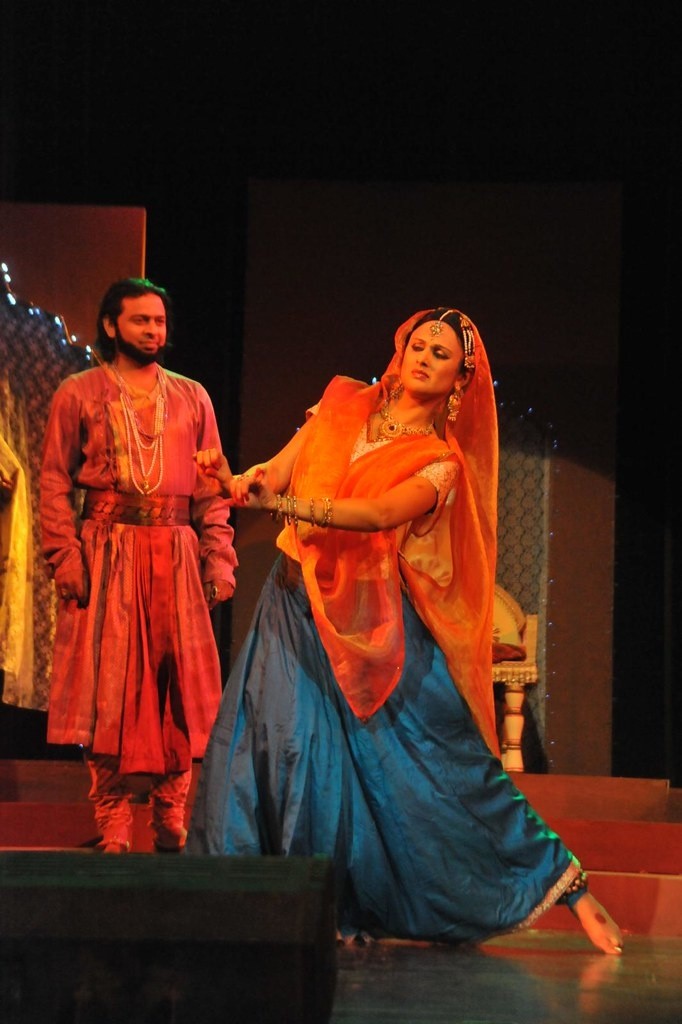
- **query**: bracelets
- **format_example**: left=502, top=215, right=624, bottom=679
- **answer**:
left=274, top=496, right=331, bottom=531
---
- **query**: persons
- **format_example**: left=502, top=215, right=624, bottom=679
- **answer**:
left=182, top=306, right=625, bottom=957
left=37, top=276, right=239, bottom=854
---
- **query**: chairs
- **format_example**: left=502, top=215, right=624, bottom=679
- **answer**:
left=492, top=581, right=537, bottom=774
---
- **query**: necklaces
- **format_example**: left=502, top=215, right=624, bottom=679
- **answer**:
left=108, top=360, right=168, bottom=497
left=376, top=394, right=433, bottom=443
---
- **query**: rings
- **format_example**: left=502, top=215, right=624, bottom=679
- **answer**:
left=210, top=586, right=221, bottom=599
left=239, top=475, right=249, bottom=479
left=61, top=588, right=68, bottom=597
